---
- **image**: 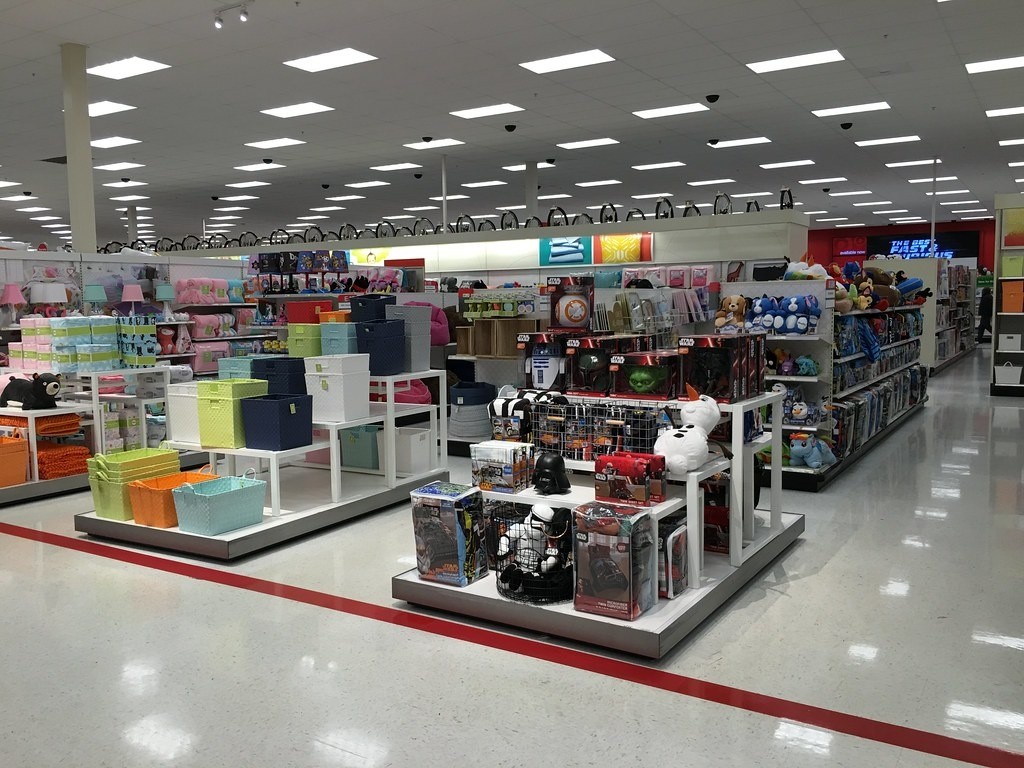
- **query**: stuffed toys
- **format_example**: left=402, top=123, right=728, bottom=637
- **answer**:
left=756, top=432, right=837, bottom=468
left=764, top=348, right=821, bottom=376
left=0, top=373, right=61, bottom=410
left=159, top=327, right=192, bottom=354
left=715, top=294, right=822, bottom=336
left=654, top=382, right=721, bottom=474
left=784, top=255, right=933, bottom=312
left=770, top=383, right=835, bottom=420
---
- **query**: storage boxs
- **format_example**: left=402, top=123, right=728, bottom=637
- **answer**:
left=384, top=258, right=425, bottom=293
left=993, top=256, right=1024, bottom=384
left=0, top=435, right=28, bottom=487
left=86, top=292, right=432, bottom=536
left=409, top=317, right=766, bottom=622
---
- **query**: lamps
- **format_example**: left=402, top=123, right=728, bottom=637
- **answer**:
left=0, top=284, right=27, bottom=330
left=121, top=285, right=145, bottom=318
left=30, top=283, right=68, bottom=317
left=247, top=250, right=348, bottom=294
left=82, top=284, right=109, bottom=316
left=155, top=284, right=176, bottom=323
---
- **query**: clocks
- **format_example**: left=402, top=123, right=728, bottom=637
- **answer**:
left=616, top=291, right=657, bottom=334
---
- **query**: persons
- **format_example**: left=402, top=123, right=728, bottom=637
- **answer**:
left=497, top=505, right=557, bottom=590
left=978, top=287, right=993, bottom=343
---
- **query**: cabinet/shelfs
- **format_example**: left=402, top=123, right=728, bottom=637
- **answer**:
left=161, top=369, right=447, bottom=517
left=709, top=281, right=928, bottom=475
left=863, top=257, right=976, bottom=376
left=458, top=391, right=785, bottom=589
left=172, top=297, right=278, bottom=377
left=989, top=193, right=1024, bottom=398
left=0, top=300, right=195, bottom=483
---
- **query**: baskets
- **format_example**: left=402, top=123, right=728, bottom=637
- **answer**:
left=0, top=426, right=28, bottom=486
left=85, top=439, right=267, bottom=535
